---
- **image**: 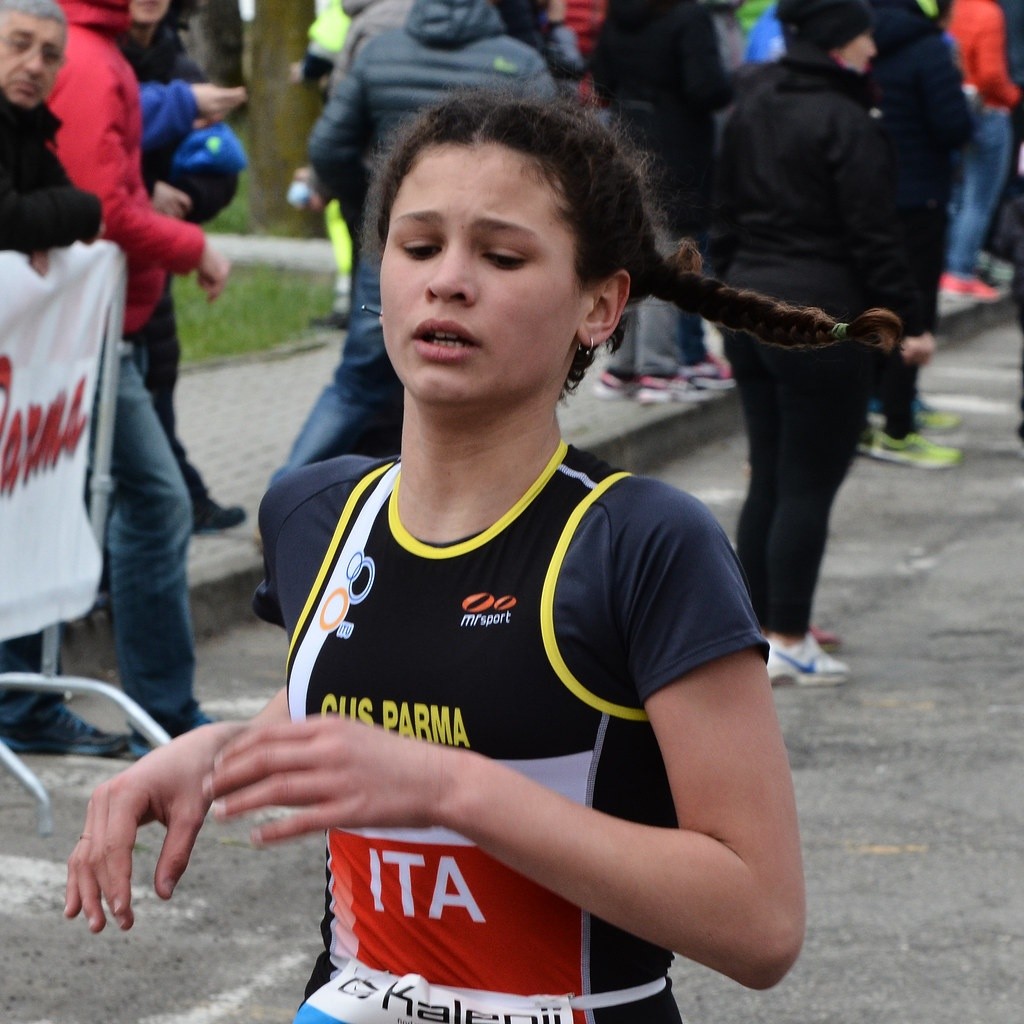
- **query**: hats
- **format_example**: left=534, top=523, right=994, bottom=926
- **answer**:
left=774, top=0, right=871, bottom=50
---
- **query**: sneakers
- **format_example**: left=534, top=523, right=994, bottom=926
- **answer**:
left=128, top=706, right=214, bottom=758
left=1, top=701, right=132, bottom=756
left=765, top=632, right=851, bottom=688
left=680, top=354, right=738, bottom=389
left=635, top=375, right=713, bottom=403
left=809, top=620, right=841, bottom=651
left=594, top=369, right=642, bottom=399
left=913, top=402, right=962, bottom=434
left=858, top=423, right=960, bottom=470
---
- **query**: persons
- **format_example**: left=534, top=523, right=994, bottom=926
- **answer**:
left=0, top=2, right=133, bottom=756
left=129, top=1, right=1022, bottom=534
left=692, top=0, right=939, bottom=686
left=60, top=91, right=904, bottom=1023
left=42, top=0, right=232, bottom=750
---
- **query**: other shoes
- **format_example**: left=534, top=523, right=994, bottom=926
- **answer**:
left=249, top=579, right=285, bottom=629
left=975, top=249, right=1017, bottom=286
left=192, top=496, right=247, bottom=534
left=939, top=273, right=999, bottom=304
